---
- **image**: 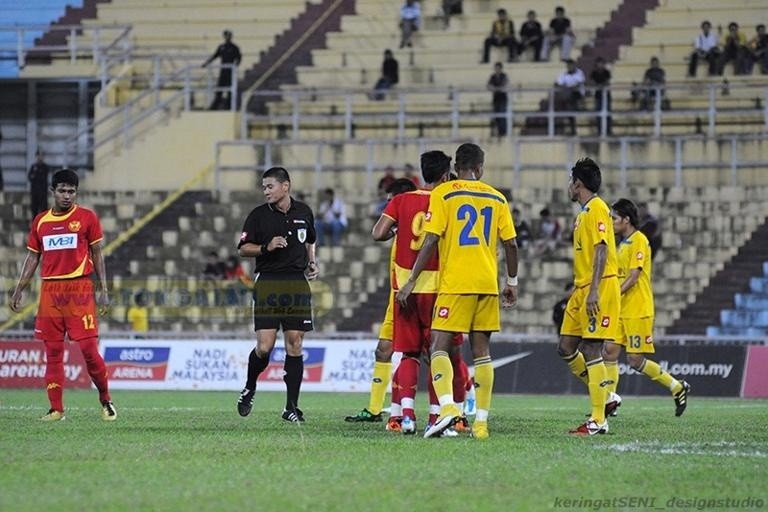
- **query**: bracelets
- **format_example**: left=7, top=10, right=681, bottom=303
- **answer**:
left=260, top=241, right=270, bottom=256
left=505, top=273, right=518, bottom=288
left=306, top=261, right=317, bottom=267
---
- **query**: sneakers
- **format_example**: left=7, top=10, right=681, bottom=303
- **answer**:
left=281, top=406, right=305, bottom=424
left=236, top=387, right=256, bottom=417
left=672, top=380, right=692, bottom=416
left=400, top=416, right=417, bottom=434
left=604, top=392, right=622, bottom=418
left=469, top=420, right=489, bottom=439
left=569, top=418, right=609, bottom=435
left=102, top=401, right=117, bottom=421
left=422, top=409, right=470, bottom=438
left=38, top=408, right=65, bottom=421
left=344, top=408, right=384, bottom=423
left=465, top=398, right=476, bottom=415
left=385, top=416, right=401, bottom=431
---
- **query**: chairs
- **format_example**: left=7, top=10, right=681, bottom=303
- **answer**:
left=0, top=182, right=768, bottom=345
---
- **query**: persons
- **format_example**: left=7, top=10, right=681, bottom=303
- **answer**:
left=395, top=142, right=522, bottom=439
left=200, top=27, right=244, bottom=112
left=200, top=248, right=225, bottom=280
left=236, top=167, right=320, bottom=424
left=127, top=288, right=156, bottom=332
left=10, top=169, right=122, bottom=424
left=370, top=149, right=473, bottom=438
left=343, top=175, right=420, bottom=423
left=600, top=198, right=692, bottom=419
left=557, top=158, right=625, bottom=436
left=458, top=357, right=477, bottom=417
left=27, top=148, right=54, bottom=220
left=553, top=280, right=577, bottom=339
left=226, top=256, right=247, bottom=281
left=315, top=0, right=768, bottom=254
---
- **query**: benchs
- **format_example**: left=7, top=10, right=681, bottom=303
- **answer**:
left=0, top=1, right=767, bottom=134
